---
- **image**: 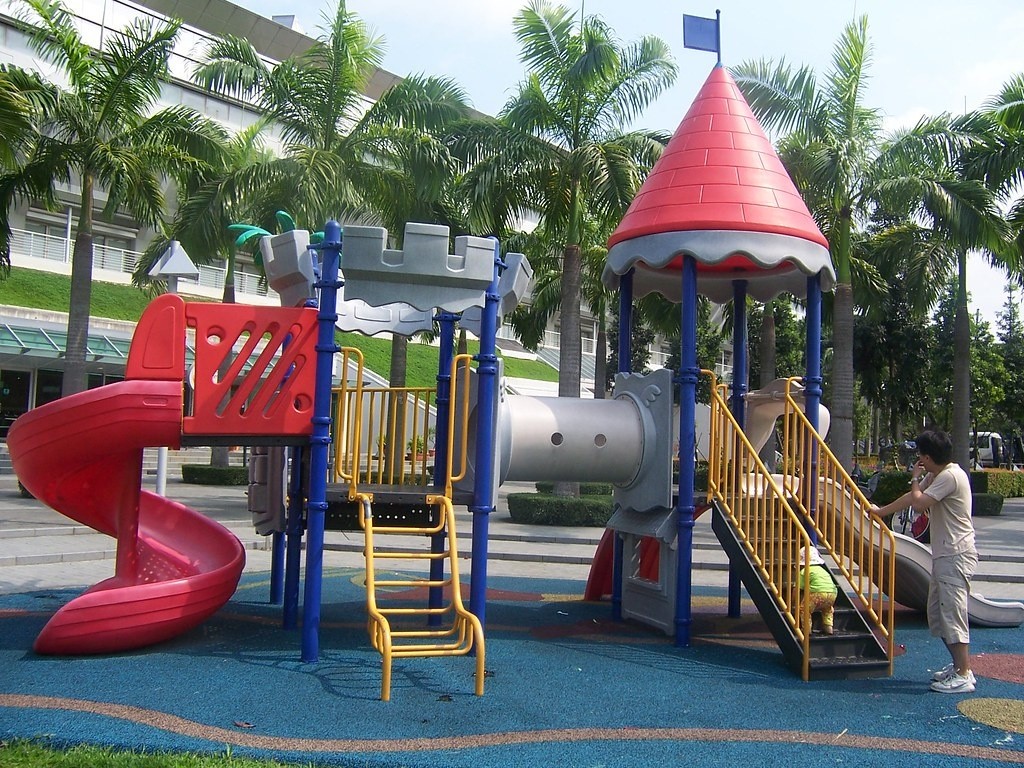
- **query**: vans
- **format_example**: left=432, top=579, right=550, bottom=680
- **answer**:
left=969, top=431, right=1003, bottom=466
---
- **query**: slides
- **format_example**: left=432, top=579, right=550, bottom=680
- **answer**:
left=733, top=468, right=1024, bottom=627
left=7, top=376, right=245, bottom=656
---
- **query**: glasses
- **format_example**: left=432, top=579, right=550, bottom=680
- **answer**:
left=916, top=452, right=930, bottom=457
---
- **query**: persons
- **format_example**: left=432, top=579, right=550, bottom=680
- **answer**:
left=791, top=545, right=838, bottom=634
left=866, top=430, right=978, bottom=693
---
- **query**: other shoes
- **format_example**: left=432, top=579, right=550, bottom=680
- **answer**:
left=822, top=626, right=833, bottom=635
left=801, top=628, right=812, bottom=635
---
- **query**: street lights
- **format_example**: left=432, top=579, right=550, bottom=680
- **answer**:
left=149, top=240, right=200, bottom=496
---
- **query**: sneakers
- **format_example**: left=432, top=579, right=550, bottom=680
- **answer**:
left=930, top=668, right=975, bottom=694
left=933, top=663, right=976, bottom=684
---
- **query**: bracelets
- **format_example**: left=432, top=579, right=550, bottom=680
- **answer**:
left=908, top=477, right=919, bottom=484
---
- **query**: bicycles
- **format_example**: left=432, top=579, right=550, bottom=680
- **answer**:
left=898, top=475, right=930, bottom=540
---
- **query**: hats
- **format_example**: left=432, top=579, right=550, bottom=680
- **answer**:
left=799, top=546, right=825, bottom=565
